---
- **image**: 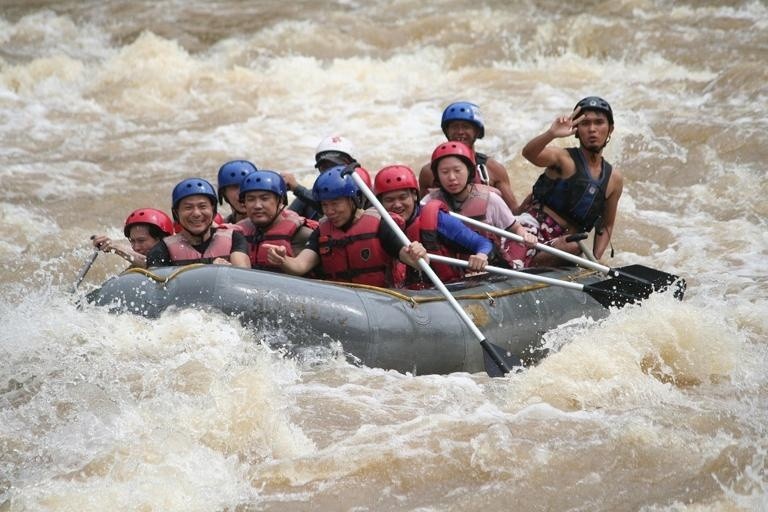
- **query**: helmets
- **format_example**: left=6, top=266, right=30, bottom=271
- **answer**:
left=431, top=141, right=477, bottom=180
left=441, top=101, right=485, bottom=138
left=218, top=134, right=371, bottom=210
left=171, top=179, right=219, bottom=222
left=573, top=97, right=613, bottom=126
left=374, top=165, right=420, bottom=204
left=123, top=209, right=173, bottom=238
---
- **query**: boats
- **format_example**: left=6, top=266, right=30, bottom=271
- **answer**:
left=74, top=258, right=689, bottom=381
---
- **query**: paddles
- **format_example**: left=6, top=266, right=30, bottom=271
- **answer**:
left=342, top=162, right=518, bottom=377
left=427, top=252, right=651, bottom=309
left=447, top=209, right=686, bottom=303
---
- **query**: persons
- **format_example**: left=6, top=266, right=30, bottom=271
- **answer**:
left=280, top=150, right=357, bottom=222
left=420, top=140, right=538, bottom=270
left=260, top=166, right=427, bottom=288
left=504, top=96, right=623, bottom=268
left=94, top=208, right=173, bottom=269
left=230, top=170, right=321, bottom=279
left=147, top=179, right=252, bottom=269
left=417, top=102, right=533, bottom=214
left=218, top=160, right=258, bottom=224
left=375, top=165, right=493, bottom=286
